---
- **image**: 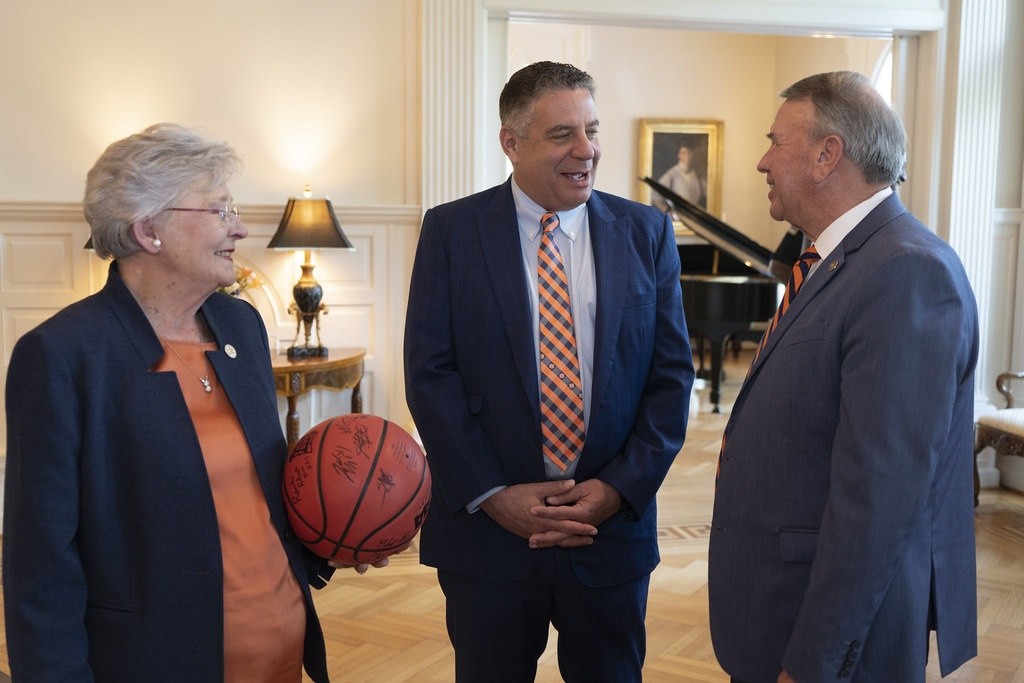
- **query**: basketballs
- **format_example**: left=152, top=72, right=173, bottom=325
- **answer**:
left=282, top=412, right=432, bottom=565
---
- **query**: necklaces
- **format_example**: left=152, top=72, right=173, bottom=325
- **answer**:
left=145, top=317, right=212, bottom=392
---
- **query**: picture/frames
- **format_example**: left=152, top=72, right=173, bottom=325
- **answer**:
left=638, top=117, right=726, bottom=237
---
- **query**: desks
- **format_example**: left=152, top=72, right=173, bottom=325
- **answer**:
left=268, top=348, right=367, bottom=458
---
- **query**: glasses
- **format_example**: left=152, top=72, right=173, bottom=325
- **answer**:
left=163, top=200, right=240, bottom=224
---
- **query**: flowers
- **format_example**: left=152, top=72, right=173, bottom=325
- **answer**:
left=217, top=267, right=265, bottom=300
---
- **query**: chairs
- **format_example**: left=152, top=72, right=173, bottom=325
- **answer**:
left=970, top=370, right=1024, bottom=508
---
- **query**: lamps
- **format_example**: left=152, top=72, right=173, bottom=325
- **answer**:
left=266, top=183, right=356, bottom=358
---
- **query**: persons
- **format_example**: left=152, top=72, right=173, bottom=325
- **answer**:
left=2, top=122, right=404, bottom=683
left=704, top=70, right=981, bottom=683
left=405, top=60, right=693, bottom=683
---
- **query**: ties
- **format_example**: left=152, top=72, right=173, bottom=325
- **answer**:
left=745, top=244, right=821, bottom=384
left=538, top=210, right=586, bottom=471
